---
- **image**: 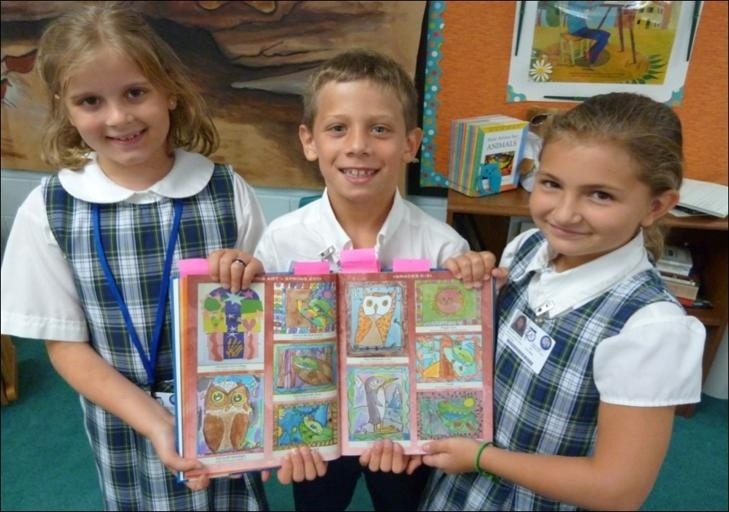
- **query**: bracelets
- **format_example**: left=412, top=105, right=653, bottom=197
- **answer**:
left=475, top=439, right=500, bottom=480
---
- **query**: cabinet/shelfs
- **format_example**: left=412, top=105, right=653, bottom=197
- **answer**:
left=442, top=187, right=729, bottom=419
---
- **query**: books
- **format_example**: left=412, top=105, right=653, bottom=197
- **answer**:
left=506, top=214, right=532, bottom=245
left=646, top=242, right=715, bottom=309
left=446, top=112, right=528, bottom=198
left=519, top=221, right=536, bottom=234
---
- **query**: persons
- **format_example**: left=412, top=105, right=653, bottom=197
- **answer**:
left=245, top=46, right=474, bottom=512
left=0, top=3, right=274, bottom=512
left=422, top=92, right=709, bottom=512
left=511, top=314, right=527, bottom=337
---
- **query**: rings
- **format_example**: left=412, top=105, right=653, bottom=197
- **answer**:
left=232, top=258, right=246, bottom=267
